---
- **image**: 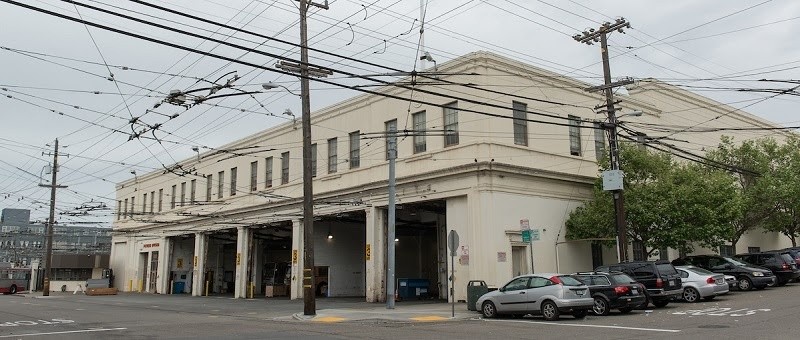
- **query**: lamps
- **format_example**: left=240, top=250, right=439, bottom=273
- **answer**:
left=327, top=232, right=334, bottom=240
left=395, top=237, right=400, bottom=242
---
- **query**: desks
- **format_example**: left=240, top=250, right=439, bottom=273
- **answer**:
left=265, top=284, right=288, bottom=298
left=398, top=278, right=433, bottom=302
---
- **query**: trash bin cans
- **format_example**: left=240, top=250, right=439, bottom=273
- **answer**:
left=467, top=281, right=489, bottom=311
left=173, top=281, right=185, bottom=293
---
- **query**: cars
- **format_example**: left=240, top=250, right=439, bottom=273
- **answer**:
left=670, top=253, right=777, bottom=291
left=568, top=269, right=650, bottom=317
left=732, top=246, right=800, bottom=287
left=672, top=264, right=730, bottom=303
left=475, top=271, right=594, bottom=321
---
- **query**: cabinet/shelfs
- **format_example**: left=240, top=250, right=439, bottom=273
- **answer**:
left=261, top=247, right=291, bottom=296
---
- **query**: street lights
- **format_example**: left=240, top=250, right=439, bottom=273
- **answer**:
left=608, top=109, right=643, bottom=264
left=262, top=82, right=317, bottom=317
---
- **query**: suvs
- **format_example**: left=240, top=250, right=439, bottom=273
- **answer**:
left=593, top=260, right=685, bottom=309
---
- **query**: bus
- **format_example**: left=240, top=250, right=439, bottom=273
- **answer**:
left=0, top=262, right=30, bottom=295
